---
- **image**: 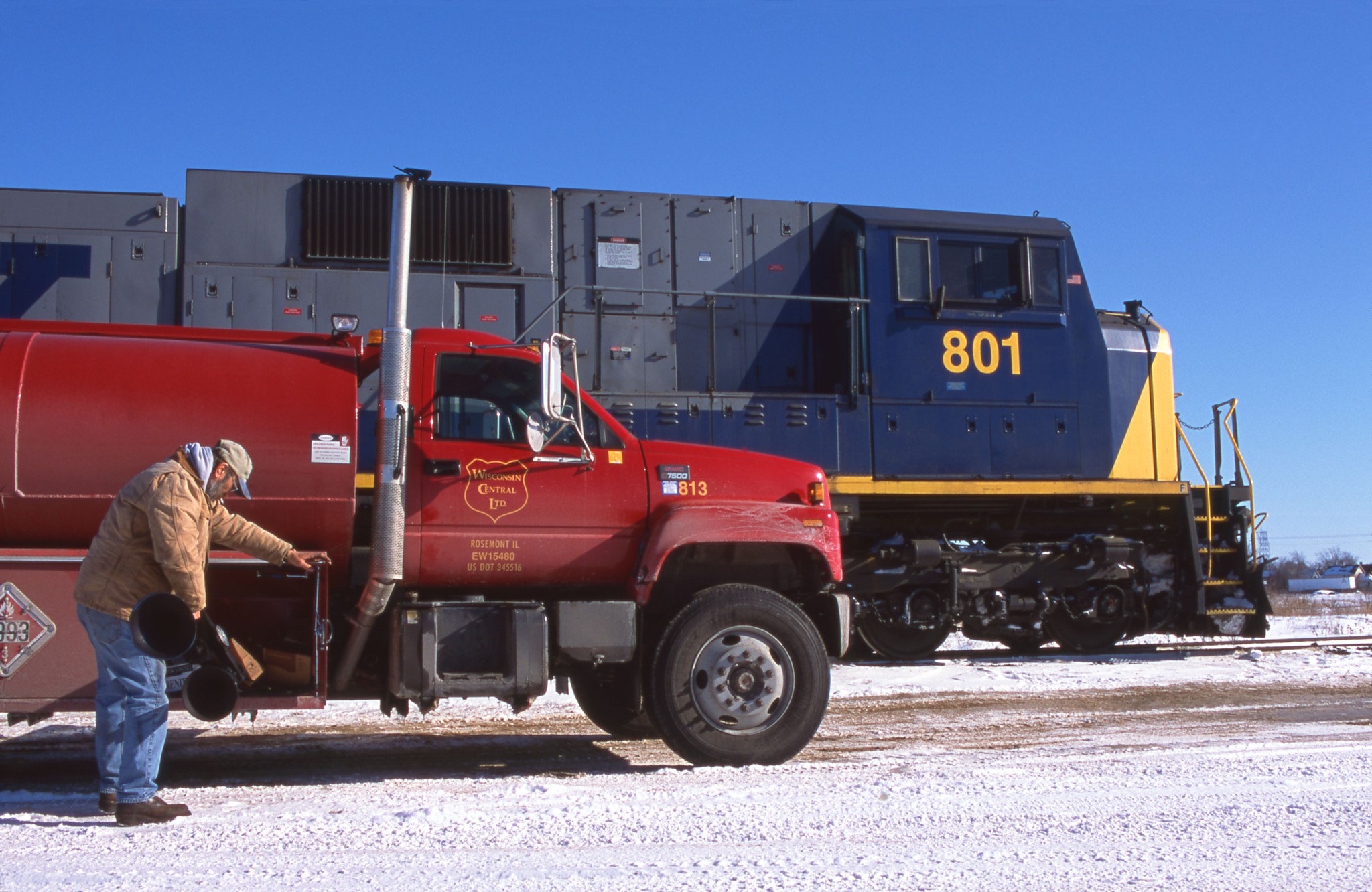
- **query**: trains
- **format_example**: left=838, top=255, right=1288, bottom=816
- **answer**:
left=0, top=166, right=1280, bottom=664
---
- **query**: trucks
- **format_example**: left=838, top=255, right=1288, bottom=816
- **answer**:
left=0, top=172, right=852, bottom=768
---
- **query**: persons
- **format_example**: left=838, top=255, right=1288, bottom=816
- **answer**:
left=72, top=438, right=333, bottom=826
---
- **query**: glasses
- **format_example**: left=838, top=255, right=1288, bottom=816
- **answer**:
left=227, top=468, right=241, bottom=492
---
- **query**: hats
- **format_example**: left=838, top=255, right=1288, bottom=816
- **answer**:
left=212, top=439, right=253, bottom=499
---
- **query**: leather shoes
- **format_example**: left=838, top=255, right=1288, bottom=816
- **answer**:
left=98, top=793, right=118, bottom=813
left=115, top=796, right=192, bottom=827
